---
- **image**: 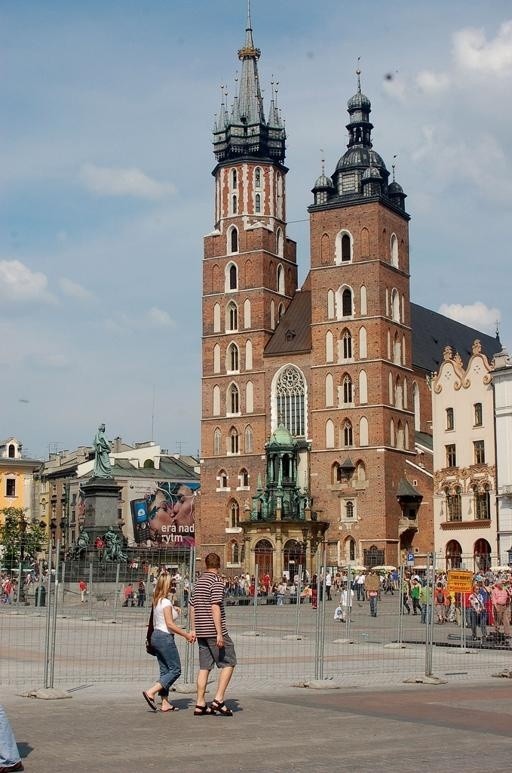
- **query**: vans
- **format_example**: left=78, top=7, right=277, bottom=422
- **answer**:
left=369, top=565, right=397, bottom=586
left=341, top=565, right=368, bottom=578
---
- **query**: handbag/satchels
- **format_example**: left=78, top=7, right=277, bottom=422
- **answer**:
left=146, top=613, right=174, bottom=655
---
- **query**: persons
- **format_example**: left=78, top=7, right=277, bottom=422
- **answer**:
left=75, top=525, right=90, bottom=550
left=173, top=483, right=201, bottom=547
left=95, top=535, right=107, bottom=560
left=221, top=560, right=512, bottom=650
left=1, top=705, right=25, bottom=772
left=92, top=422, right=116, bottom=479
left=189, top=553, right=237, bottom=716
left=139, top=485, right=176, bottom=548
left=0, top=558, right=204, bottom=609
left=104, top=525, right=128, bottom=562
left=142, top=570, right=192, bottom=711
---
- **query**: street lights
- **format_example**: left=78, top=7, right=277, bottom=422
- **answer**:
left=16, top=513, right=30, bottom=602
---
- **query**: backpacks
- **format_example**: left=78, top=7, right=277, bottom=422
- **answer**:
left=436, top=588, right=445, bottom=603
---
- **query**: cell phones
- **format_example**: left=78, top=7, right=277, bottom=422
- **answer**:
left=130, top=498, right=151, bottom=543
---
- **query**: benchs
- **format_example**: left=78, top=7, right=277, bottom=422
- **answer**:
left=223, top=595, right=310, bottom=606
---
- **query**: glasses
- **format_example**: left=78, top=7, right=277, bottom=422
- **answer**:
left=160, top=705, right=179, bottom=712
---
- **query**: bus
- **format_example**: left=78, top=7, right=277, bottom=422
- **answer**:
left=2, top=569, right=34, bottom=583
left=411, top=565, right=435, bottom=579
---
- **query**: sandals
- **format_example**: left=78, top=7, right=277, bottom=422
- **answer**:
left=194, top=699, right=233, bottom=715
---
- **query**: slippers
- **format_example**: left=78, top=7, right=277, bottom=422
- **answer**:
left=142, top=690, right=158, bottom=711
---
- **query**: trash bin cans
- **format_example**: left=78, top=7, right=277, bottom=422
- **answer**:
left=35, top=586, right=46, bottom=607
